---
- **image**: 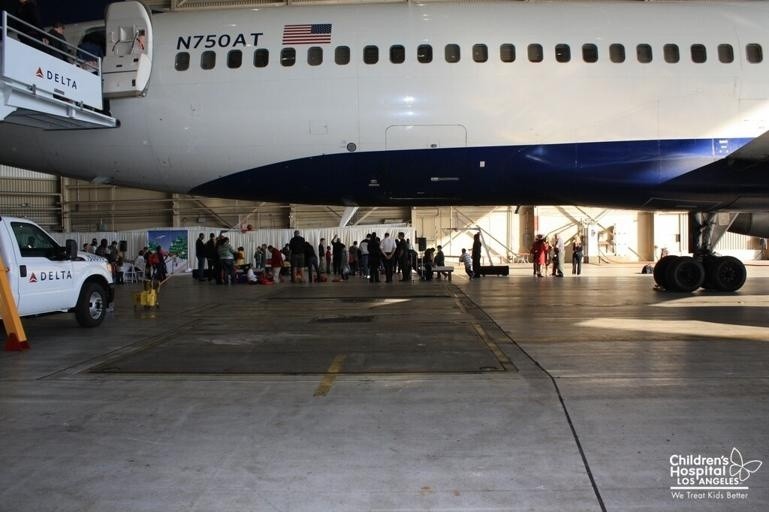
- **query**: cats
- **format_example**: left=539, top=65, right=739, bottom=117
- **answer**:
left=0, top=1, right=769, bottom=292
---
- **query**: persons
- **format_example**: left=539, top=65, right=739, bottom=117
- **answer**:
left=196, top=230, right=448, bottom=287
left=459, top=248, right=473, bottom=279
left=471, top=233, right=481, bottom=278
left=82, top=238, right=167, bottom=286
left=531, top=234, right=583, bottom=277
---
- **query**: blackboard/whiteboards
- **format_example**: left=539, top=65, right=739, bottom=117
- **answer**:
left=0, top=218, right=117, bottom=327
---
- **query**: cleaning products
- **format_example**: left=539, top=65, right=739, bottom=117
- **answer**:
left=131, top=280, right=160, bottom=311
